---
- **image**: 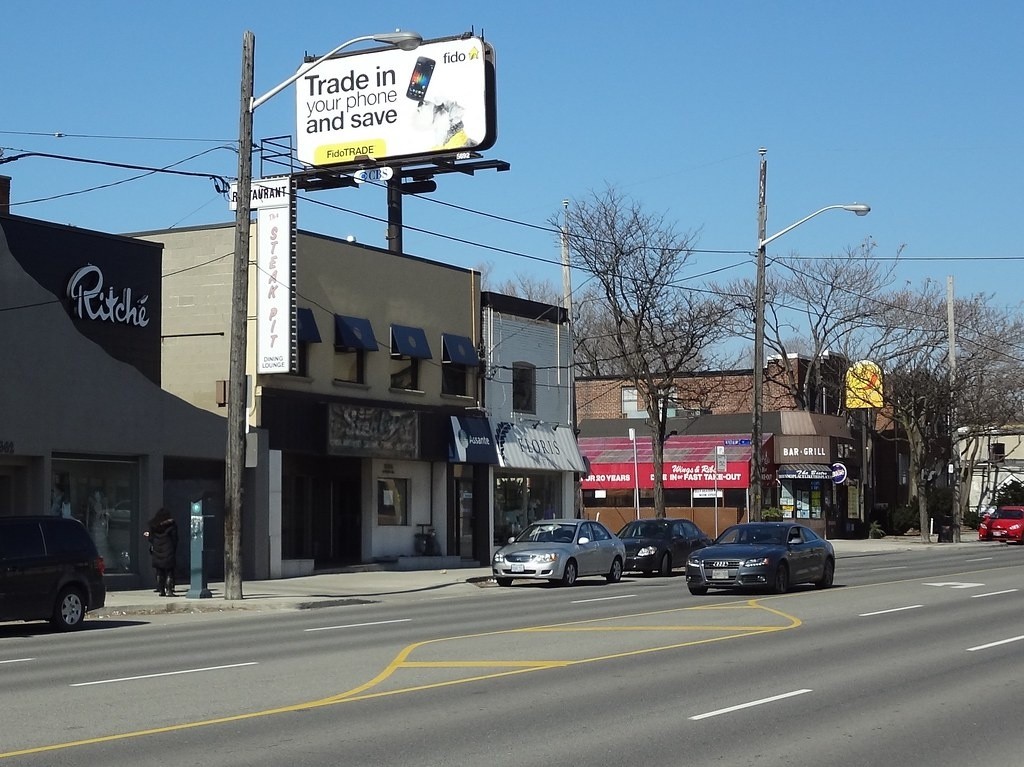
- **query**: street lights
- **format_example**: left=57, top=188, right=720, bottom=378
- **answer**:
left=751, top=203, right=871, bottom=523
left=223, top=30, right=425, bottom=593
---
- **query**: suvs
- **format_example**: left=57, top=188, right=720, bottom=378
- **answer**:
left=1, top=514, right=107, bottom=632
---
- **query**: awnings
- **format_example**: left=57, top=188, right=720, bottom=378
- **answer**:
left=577, top=433, right=775, bottom=489
left=488, top=415, right=587, bottom=471
left=779, top=464, right=832, bottom=479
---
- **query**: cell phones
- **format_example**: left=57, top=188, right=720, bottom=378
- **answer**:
left=407, top=56, right=435, bottom=101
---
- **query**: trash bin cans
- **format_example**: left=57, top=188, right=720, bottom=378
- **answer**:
left=413, top=534, right=433, bottom=554
left=938, top=512, right=954, bottom=542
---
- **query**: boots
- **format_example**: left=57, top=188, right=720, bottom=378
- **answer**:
left=165, top=577, right=174, bottom=597
left=157, top=576, right=165, bottom=596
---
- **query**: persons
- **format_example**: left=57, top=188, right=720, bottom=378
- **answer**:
left=148, top=510, right=178, bottom=597
left=49, top=484, right=68, bottom=515
left=86, top=487, right=114, bottom=570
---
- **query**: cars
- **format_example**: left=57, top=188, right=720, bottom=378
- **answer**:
left=978, top=506, right=1024, bottom=545
left=492, top=518, right=627, bottom=586
left=685, top=521, right=835, bottom=596
left=614, top=518, right=715, bottom=578
